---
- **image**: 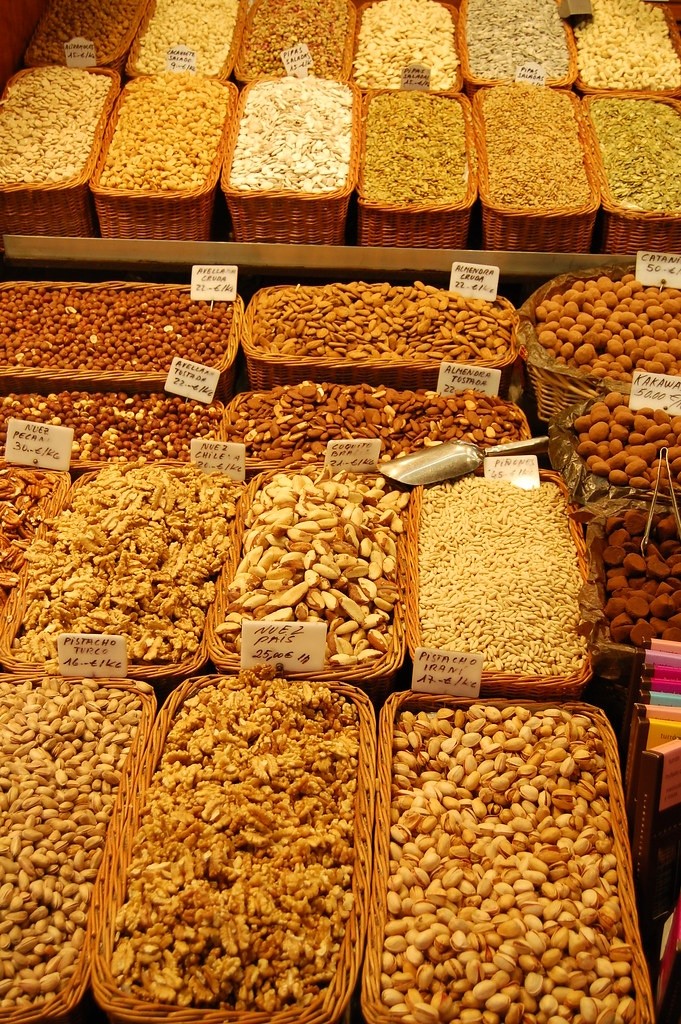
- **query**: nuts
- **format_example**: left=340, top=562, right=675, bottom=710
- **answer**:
left=0, top=268, right=678, bottom=1024
left=0, top=0, right=681, bottom=212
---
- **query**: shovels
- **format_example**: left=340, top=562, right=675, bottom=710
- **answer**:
left=375, top=433, right=550, bottom=487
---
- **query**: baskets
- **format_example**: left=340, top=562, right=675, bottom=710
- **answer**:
left=0, top=0, right=681, bottom=253
left=2, top=271, right=681, bottom=700
left=88, top=673, right=376, bottom=1024
left=0, top=675, right=157, bottom=1024
left=360, top=690, right=655, bottom=1024
